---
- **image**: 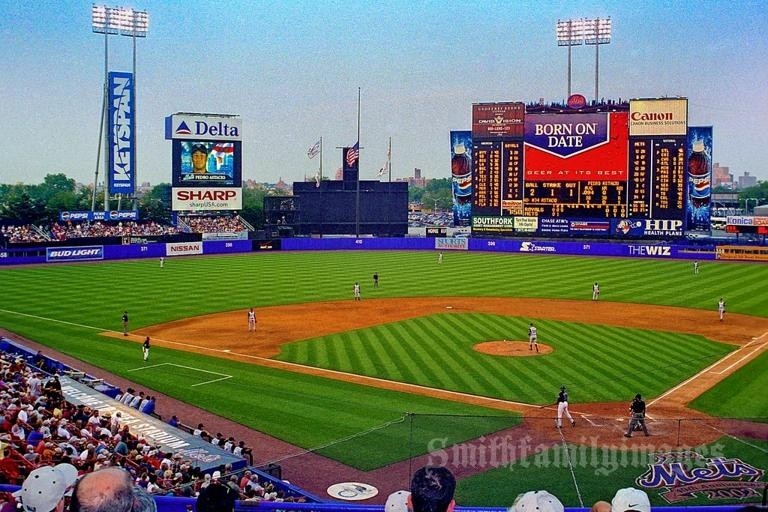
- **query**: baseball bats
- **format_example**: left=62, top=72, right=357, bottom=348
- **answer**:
left=541, top=403, right=557, bottom=409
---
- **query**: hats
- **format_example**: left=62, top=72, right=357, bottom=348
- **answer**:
left=11, top=461, right=79, bottom=512
left=560, top=386, right=565, bottom=389
left=1, top=353, right=307, bottom=503
left=636, top=394, right=641, bottom=399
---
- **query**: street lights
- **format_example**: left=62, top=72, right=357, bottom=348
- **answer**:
left=430, top=197, right=441, bottom=214
left=92, top=2, right=147, bottom=215
left=557, top=16, right=610, bottom=105
left=739, top=197, right=765, bottom=216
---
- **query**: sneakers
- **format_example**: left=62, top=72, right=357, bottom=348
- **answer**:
left=555, top=426, right=562, bottom=428
left=624, top=434, right=632, bottom=437
left=645, top=434, right=649, bottom=436
left=572, top=422, right=575, bottom=427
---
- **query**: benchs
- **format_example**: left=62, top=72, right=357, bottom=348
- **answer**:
left=0, top=338, right=320, bottom=511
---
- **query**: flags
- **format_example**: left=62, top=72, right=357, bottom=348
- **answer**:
left=346, top=142, right=359, bottom=168
left=375, top=140, right=391, bottom=179
left=307, top=141, right=320, bottom=159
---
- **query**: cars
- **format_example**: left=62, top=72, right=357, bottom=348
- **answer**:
left=408, top=212, right=455, bottom=227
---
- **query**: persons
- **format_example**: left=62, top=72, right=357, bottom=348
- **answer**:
left=248, top=308, right=257, bottom=332
left=591, top=500, right=612, bottom=512
left=159, top=256, right=165, bottom=268
left=555, top=385, right=577, bottom=429
left=1, top=348, right=305, bottom=512
left=191, top=143, right=209, bottom=173
left=628, top=399, right=643, bottom=430
left=2, top=213, right=245, bottom=243
left=624, top=393, right=649, bottom=438
left=592, top=282, right=599, bottom=301
left=407, top=466, right=456, bottom=512
left=527, top=323, right=539, bottom=353
left=353, top=281, right=362, bottom=304
left=437, top=251, right=444, bottom=265
left=121, top=310, right=129, bottom=336
left=693, top=260, right=699, bottom=274
left=373, top=272, right=379, bottom=289
left=142, top=336, right=150, bottom=361
left=717, top=298, right=726, bottom=322
